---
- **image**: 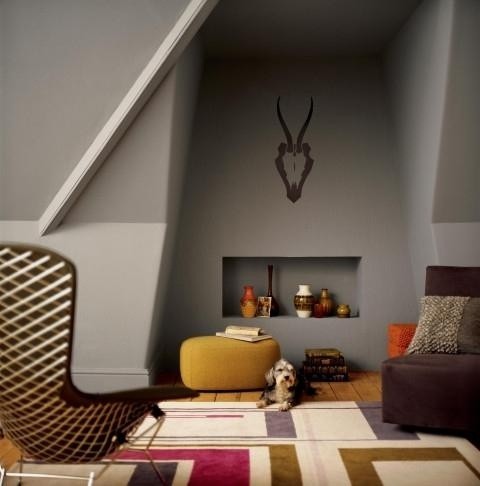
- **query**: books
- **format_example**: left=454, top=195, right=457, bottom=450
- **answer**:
left=305, top=354, right=345, bottom=366
left=303, top=360, right=348, bottom=374
left=299, top=367, right=348, bottom=381
left=215, top=330, right=273, bottom=343
left=226, top=323, right=262, bottom=336
left=305, top=347, right=341, bottom=357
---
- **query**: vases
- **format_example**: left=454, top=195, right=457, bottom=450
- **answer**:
left=240, top=265, right=350, bottom=318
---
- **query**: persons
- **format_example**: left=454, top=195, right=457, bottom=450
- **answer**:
left=256, top=298, right=270, bottom=316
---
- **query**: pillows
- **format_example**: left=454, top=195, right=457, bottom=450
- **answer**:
left=405, top=296, right=480, bottom=354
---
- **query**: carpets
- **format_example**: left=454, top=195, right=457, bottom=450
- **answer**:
left=2, top=402, right=480, bottom=486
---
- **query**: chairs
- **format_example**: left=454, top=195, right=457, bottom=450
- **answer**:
left=0, top=244, right=200, bottom=486
left=382, top=267, right=480, bottom=433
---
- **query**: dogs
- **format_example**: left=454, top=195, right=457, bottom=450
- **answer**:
left=255, top=356, right=322, bottom=412
